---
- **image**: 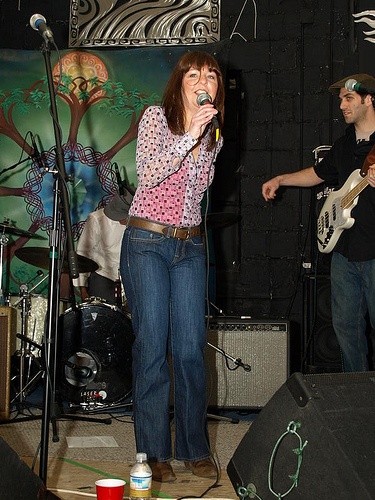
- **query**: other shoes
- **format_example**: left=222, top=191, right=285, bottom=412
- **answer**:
left=149, top=462, right=177, bottom=481
left=184, top=459, right=218, bottom=477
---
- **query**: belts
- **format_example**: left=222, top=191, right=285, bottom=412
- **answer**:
left=128, top=216, right=200, bottom=239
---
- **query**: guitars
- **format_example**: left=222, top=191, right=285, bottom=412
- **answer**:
left=317, top=168, right=369, bottom=253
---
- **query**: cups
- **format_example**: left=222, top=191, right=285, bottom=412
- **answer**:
left=95, top=479, right=126, bottom=500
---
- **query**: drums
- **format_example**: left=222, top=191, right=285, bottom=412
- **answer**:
left=49, top=300, right=134, bottom=408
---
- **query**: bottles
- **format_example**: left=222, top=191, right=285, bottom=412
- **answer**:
left=129, top=452, right=152, bottom=500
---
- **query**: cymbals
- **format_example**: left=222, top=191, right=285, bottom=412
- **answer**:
left=0, top=222, right=46, bottom=240
left=14, top=247, right=99, bottom=273
left=201, top=212, right=242, bottom=229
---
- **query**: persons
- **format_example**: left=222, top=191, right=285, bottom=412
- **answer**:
left=261, top=75, right=375, bottom=373
left=119, top=50, right=227, bottom=485
left=72, top=188, right=139, bottom=320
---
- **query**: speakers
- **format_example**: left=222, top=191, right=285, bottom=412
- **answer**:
left=224, top=370, right=375, bottom=499
left=0, top=434, right=63, bottom=500
left=301, top=275, right=347, bottom=372
left=0, top=306, right=17, bottom=421
left=164, top=318, right=290, bottom=411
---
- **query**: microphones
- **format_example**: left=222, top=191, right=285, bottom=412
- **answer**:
left=345, top=79, right=375, bottom=98
left=30, top=132, right=43, bottom=168
left=196, top=92, right=218, bottom=130
left=29, top=13, right=53, bottom=42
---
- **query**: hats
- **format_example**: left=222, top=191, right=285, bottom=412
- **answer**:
left=329, top=73, right=375, bottom=95
left=104, top=187, right=135, bottom=221
